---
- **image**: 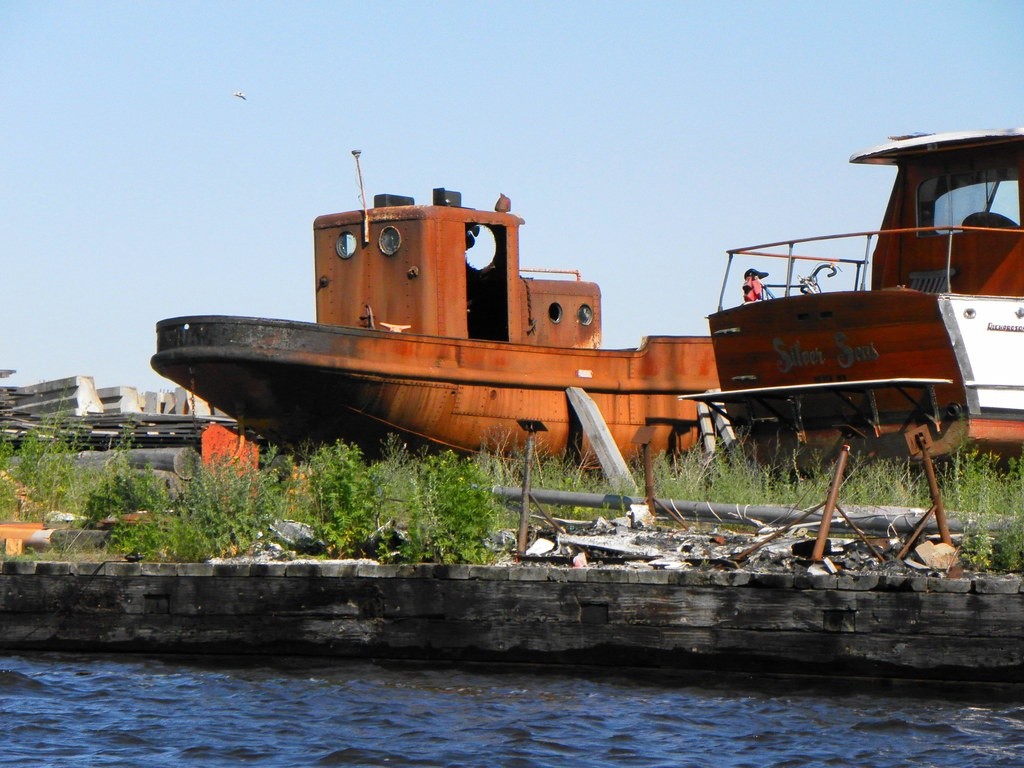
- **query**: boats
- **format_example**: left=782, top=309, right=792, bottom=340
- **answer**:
left=669, top=128, right=1023, bottom=486
left=149, top=149, right=719, bottom=477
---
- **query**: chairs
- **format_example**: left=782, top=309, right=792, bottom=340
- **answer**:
left=908, top=267, right=957, bottom=294
